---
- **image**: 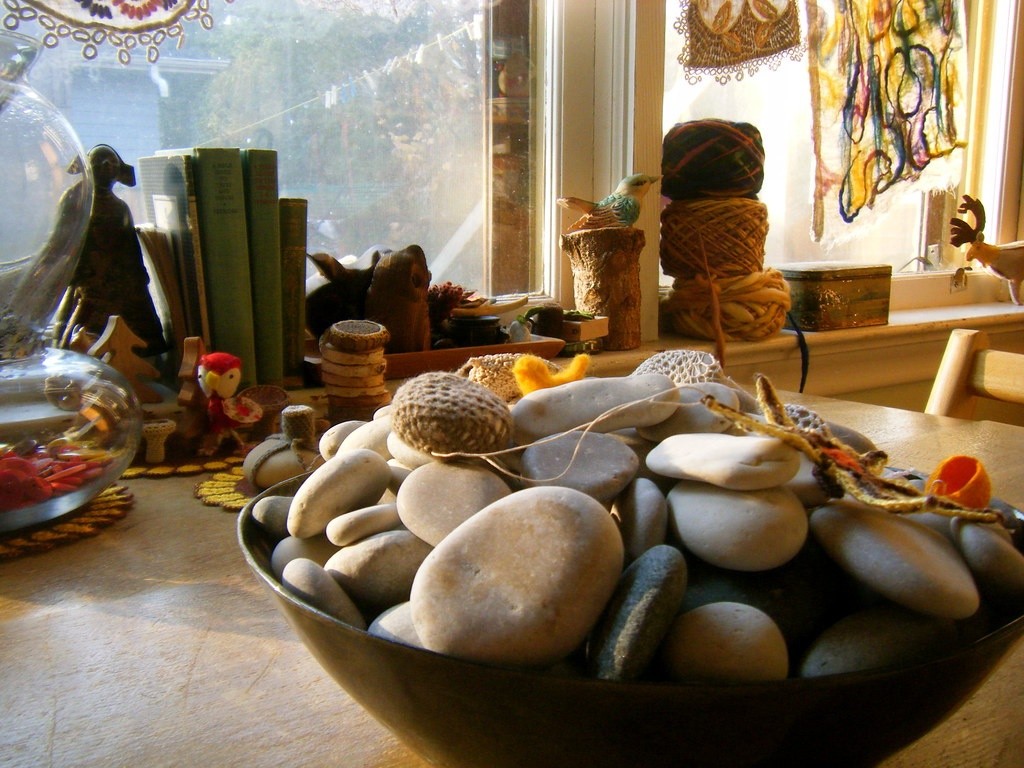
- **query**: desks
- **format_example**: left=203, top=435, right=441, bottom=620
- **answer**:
left=0, top=381, right=1023, bottom=767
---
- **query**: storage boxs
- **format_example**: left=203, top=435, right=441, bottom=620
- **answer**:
left=773, top=261, right=892, bottom=332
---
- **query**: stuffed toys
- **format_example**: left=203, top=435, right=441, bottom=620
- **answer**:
left=196, top=353, right=263, bottom=454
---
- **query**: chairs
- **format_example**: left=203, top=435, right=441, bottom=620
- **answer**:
left=924, top=328, right=1024, bottom=427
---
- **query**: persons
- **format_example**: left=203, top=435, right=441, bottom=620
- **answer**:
left=52, top=144, right=168, bottom=355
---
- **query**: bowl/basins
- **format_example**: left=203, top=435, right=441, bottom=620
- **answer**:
left=236, top=466, right=1024, bottom=768
